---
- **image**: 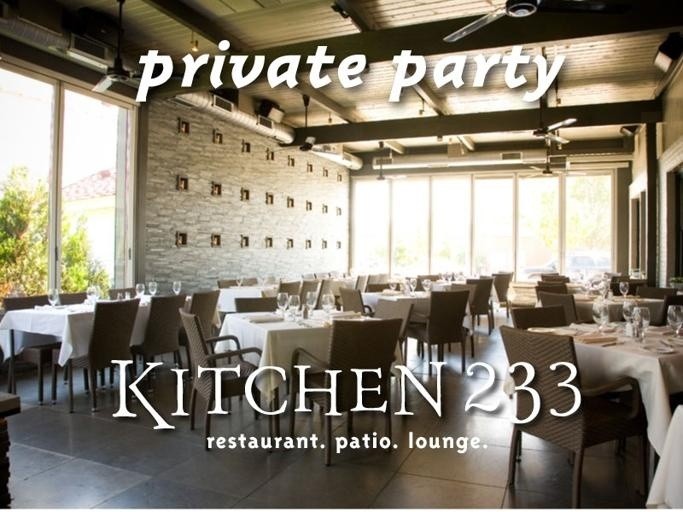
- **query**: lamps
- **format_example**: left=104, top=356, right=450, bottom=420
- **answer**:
left=620, top=126, right=638, bottom=137
left=191, top=40, right=199, bottom=52
left=654, top=32, right=683, bottom=73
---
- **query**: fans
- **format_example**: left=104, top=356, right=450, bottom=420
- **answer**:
left=270, top=95, right=339, bottom=155
left=361, top=142, right=407, bottom=180
left=49, top=0, right=183, bottom=95
left=530, top=150, right=568, bottom=175
left=513, top=97, right=577, bottom=145
left=443, top=0, right=604, bottom=44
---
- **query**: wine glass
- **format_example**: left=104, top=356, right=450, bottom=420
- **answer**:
left=389, top=278, right=431, bottom=297
left=48, top=288, right=59, bottom=310
left=593, top=282, right=683, bottom=343
left=172, top=280, right=181, bottom=296
left=86, top=286, right=101, bottom=305
left=277, top=292, right=334, bottom=323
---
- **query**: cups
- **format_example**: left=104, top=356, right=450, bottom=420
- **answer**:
left=136, top=281, right=157, bottom=295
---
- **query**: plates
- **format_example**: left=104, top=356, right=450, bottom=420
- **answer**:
left=641, top=345, right=675, bottom=355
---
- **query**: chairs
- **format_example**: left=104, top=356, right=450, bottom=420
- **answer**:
left=0, top=272, right=683, bottom=509
